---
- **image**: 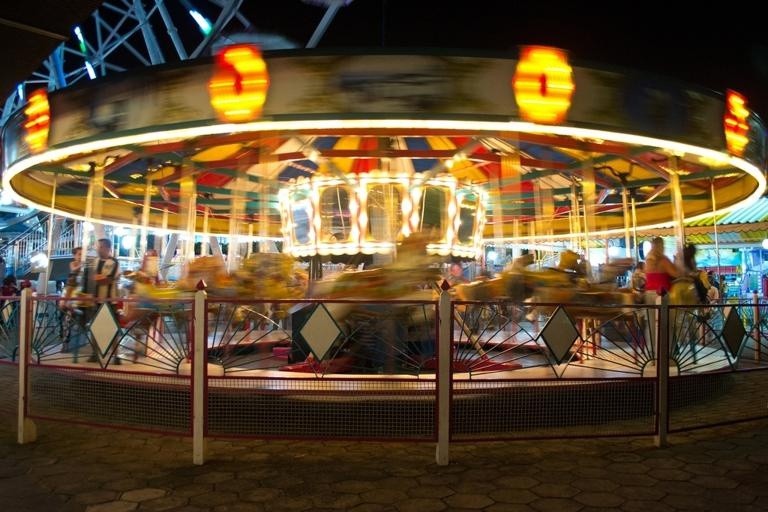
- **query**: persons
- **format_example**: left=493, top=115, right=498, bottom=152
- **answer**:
left=576, top=239, right=727, bottom=303
left=0, top=238, right=123, bottom=364
left=443, top=256, right=470, bottom=286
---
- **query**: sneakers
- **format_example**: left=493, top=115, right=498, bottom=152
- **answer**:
left=87, top=352, right=98, bottom=362
left=112, top=356, right=121, bottom=364
